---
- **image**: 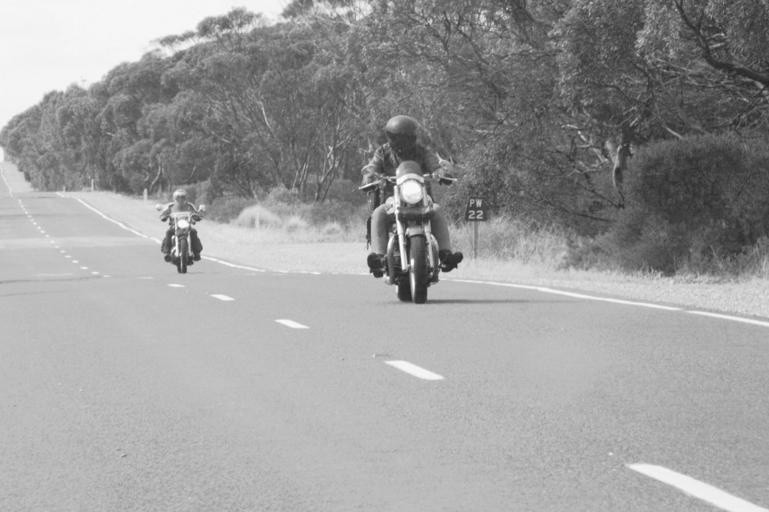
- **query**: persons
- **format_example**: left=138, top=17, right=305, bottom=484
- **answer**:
left=361, top=115, right=462, bottom=278
left=160, top=188, right=202, bottom=261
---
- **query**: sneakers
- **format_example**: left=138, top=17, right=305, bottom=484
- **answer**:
left=194, top=253, right=201, bottom=260
left=165, top=254, right=171, bottom=261
left=440, top=249, right=463, bottom=270
left=368, top=253, right=383, bottom=277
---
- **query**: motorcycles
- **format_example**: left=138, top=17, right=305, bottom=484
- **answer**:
left=155, top=202, right=206, bottom=274
left=357, top=157, right=458, bottom=305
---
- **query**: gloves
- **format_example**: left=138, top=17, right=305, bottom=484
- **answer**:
left=193, top=215, right=201, bottom=220
left=161, top=216, right=167, bottom=221
left=361, top=165, right=380, bottom=191
left=436, top=160, right=453, bottom=184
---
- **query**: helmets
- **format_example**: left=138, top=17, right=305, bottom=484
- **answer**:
left=385, top=115, right=419, bottom=152
left=174, top=189, right=188, bottom=207
